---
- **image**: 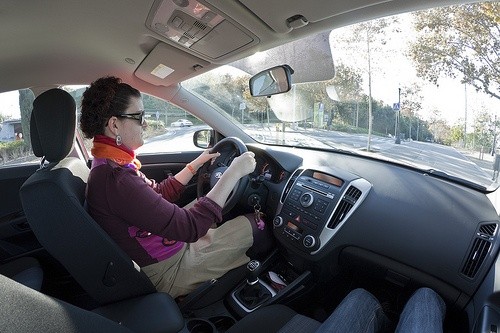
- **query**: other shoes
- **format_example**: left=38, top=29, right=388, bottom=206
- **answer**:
left=377, top=289, right=393, bottom=321
left=394, top=293, right=406, bottom=324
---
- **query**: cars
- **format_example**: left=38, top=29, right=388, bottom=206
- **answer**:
left=170, top=119, right=194, bottom=127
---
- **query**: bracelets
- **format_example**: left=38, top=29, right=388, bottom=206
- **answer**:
left=186, top=164, right=197, bottom=175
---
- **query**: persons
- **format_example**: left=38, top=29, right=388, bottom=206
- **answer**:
left=316, top=287, right=446, bottom=333
left=80, top=76, right=274, bottom=301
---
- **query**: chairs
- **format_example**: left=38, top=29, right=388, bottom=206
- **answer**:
left=0, top=274, right=332, bottom=333
left=18, top=88, right=157, bottom=303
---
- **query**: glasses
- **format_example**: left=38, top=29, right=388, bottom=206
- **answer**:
left=105, top=111, right=145, bottom=127
